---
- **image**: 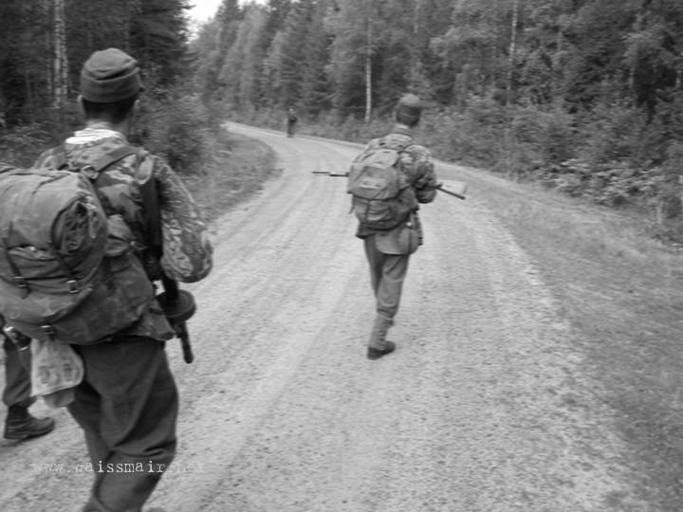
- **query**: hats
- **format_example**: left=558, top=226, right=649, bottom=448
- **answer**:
left=394, top=94, right=421, bottom=122
left=79, top=47, right=146, bottom=104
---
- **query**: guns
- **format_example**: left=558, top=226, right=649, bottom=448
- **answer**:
left=142, top=181, right=196, bottom=363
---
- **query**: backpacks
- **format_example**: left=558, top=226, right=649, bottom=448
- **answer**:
left=0, top=142, right=176, bottom=348
left=346, top=137, right=419, bottom=230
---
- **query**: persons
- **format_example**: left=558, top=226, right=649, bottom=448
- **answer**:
left=283, top=109, right=297, bottom=138
left=0, top=314, right=55, bottom=443
left=347, top=92, right=439, bottom=362
left=21, top=44, right=216, bottom=511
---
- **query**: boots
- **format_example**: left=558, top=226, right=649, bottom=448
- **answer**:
left=4, top=405, right=55, bottom=440
left=368, top=312, right=395, bottom=359
